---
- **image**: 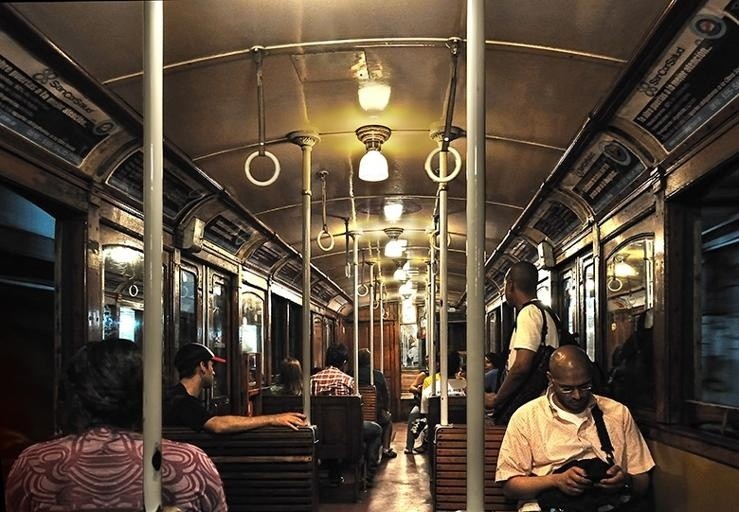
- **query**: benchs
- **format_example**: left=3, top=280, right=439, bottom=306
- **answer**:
left=358, top=385, right=378, bottom=423
left=427, top=396, right=466, bottom=456
left=164, top=425, right=319, bottom=512
left=432, top=424, right=512, bottom=512
left=262, top=393, right=368, bottom=504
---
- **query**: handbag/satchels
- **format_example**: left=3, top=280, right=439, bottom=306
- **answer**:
left=494, top=344, right=557, bottom=427
left=559, top=331, right=578, bottom=345
left=536, top=458, right=620, bottom=512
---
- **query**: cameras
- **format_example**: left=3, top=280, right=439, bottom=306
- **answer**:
left=585, top=458, right=611, bottom=482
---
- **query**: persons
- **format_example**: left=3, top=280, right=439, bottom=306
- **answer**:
left=495, top=345, right=656, bottom=512
left=308, top=343, right=383, bottom=486
left=404, top=334, right=500, bottom=455
left=264, top=357, right=303, bottom=396
left=5, top=339, right=229, bottom=512
left=310, top=368, right=323, bottom=376
left=345, top=348, right=396, bottom=459
left=162, top=344, right=307, bottom=434
left=484, top=261, right=563, bottom=424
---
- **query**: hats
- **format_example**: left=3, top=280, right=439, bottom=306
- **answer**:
left=174, top=343, right=227, bottom=370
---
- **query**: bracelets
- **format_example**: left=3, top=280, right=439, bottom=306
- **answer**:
left=621, top=475, right=633, bottom=495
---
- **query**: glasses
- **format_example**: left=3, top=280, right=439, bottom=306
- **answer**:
left=553, top=384, right=592, bottom=393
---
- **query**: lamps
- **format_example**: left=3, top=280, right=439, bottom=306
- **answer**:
left=392, top=258, right=408, bottom=281
left=355, top=124, right=391, bottom=183
left=383, top=227, right=404, bottom=258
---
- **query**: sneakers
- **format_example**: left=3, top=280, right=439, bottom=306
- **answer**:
left=382, top=452, right=397, bottom=458
